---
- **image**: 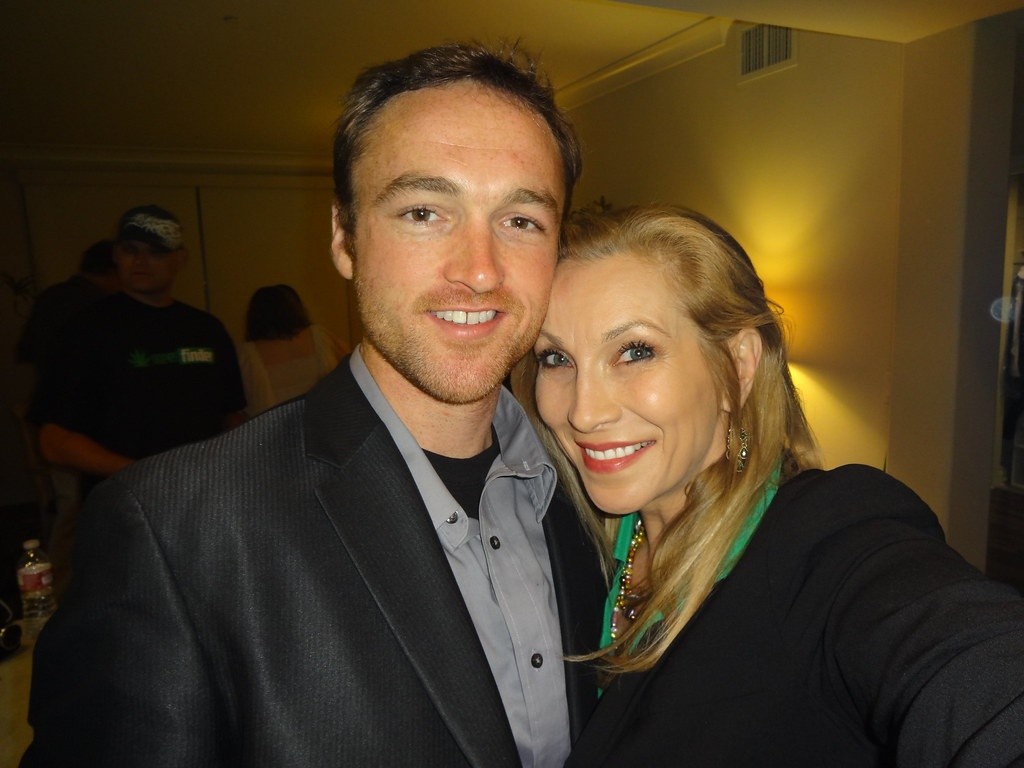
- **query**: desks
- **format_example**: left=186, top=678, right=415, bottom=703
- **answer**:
left=0, top=618, right=50, bottom=768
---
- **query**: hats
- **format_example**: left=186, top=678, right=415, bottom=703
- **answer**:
left=119, top=206, right=183, bottom=254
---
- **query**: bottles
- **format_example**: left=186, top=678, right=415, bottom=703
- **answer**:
left=16, top=539, right=57, bottom=644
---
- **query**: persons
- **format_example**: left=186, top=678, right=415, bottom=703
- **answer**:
left=19, top=45, right=607, bottom=767
left=512, top=202, right=1024, bottom=768
left=235, top=283, right=346, bottom=419
left=12, top=206, right=249, bottom=608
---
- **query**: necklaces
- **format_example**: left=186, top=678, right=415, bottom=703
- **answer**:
left=608, top=522, right=657, bottom=657
